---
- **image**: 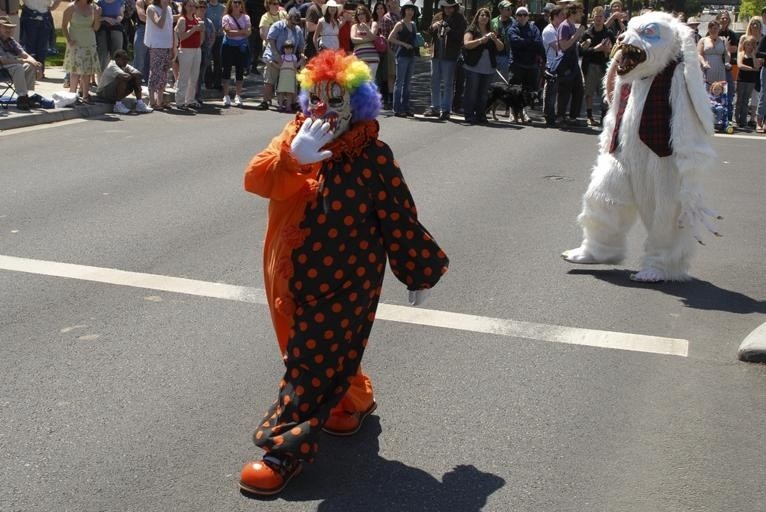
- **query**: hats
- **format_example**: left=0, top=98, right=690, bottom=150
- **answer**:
left=401, top=2, right=421, bottom=18
left=516, top=7, right=529, bottom=14
left=686, top=16, right=700, bottom=26
left=442, top=0, right=461, bottom=7
left=543, top=3, right=556, bottom=10
left=322, top=0, right=344, bottom=15
left=498, top=1, right=513, bottom=8
left=114, top=49, right=130, bottom=60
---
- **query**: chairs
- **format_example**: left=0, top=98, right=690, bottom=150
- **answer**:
left=1, top=60, right=19, bottom=110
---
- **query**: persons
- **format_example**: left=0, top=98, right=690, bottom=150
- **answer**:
left=2, top=2, right=766, bottom=133
left=238, top=46, right=453, bottom=498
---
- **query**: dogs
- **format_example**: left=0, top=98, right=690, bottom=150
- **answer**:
left=483, top=85, right=543, bottom=125
left=495, top=82, right=532, bottom=122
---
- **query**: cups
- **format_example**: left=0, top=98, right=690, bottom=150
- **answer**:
left=198, top=20, right=204, bottom=24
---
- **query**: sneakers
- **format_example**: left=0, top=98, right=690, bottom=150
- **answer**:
left=234, top=97, right=242, bottom=107
left=136, top=102, right=153, bottom=113
left=48, top=48, right=58, bottom=55
left=176, top=96, right=203, bottom=113
left=63, top=79, right=98, bottom=106
left=423, top=107, right=489, bottom=127
left=510, top=111, right=531, bottom=124
left=0, top=16, right=17, bottom=27
left=545, top=114, right=601, bottom=127
left=256, top=100, right=292, bottom=113
left=113, top=103, right=131, bottom=114
left=147, top=102, right=171, bottom=111
left=243, top=67, right=258, bottom=75
left=224, top=96, right=231, bottom=106
left=383, top=102, right=414, bottom=118
left=16, top=94, right=40, bottom=111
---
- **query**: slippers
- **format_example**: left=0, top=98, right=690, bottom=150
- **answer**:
left=736, top=120, right=765, bottom=133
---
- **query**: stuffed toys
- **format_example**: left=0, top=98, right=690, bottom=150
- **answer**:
left=560, top=10, right=725, bottom=285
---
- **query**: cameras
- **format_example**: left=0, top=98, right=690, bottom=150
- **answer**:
left=619, top=12, right=628, bottom=20
left=440, top=21, right=448, bottom=37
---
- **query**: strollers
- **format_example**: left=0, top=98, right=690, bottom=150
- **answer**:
left=706, top=79, right=734, bottom=134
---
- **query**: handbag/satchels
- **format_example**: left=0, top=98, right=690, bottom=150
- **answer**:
left=416, top=32, right=426, bottom=46
left=544, top=56, right=565, bottom=83
left=240, top=40, right=252, bottom=58
left=374, top=35, right=388, bottom=51
left=456, top=41, right=468, bottom=65
